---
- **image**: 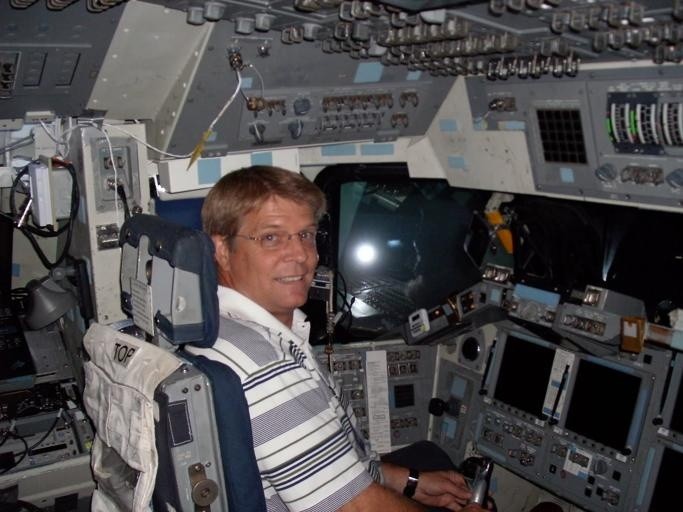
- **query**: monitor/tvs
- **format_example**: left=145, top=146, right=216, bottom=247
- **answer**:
left=487, top=332, right=556, bottom=420
left=564, top=359, right=642, bottom=454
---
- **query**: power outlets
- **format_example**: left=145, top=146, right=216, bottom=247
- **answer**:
left=25, top=111, right=56, bottom=123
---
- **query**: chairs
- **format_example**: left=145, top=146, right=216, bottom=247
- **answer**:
left=79, top=215, right=266, bottom=512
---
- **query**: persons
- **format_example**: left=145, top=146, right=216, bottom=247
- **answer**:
left=179, top=164, right=496, bottom=511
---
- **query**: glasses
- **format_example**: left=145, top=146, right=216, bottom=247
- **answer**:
left=228, top=229, right=316, bottom=247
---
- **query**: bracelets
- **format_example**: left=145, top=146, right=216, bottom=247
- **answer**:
left=401, top=465, right=420, bottom=500
